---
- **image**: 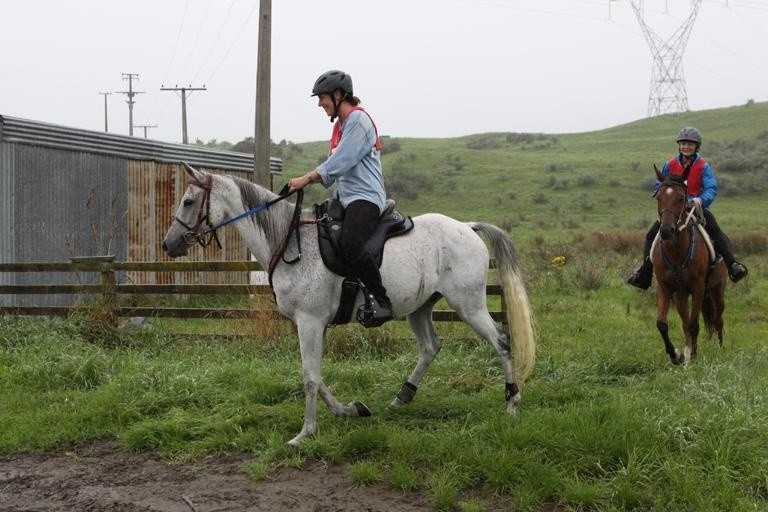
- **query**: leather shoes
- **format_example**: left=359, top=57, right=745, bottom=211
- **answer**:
left=363, top=295, right=392, bottom=325
left=729, top=262, right=745, bottom=277
left=628, top=272, right=650, bottom=290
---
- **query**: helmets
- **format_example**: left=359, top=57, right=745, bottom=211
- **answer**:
left=310, top=70, right=352, bottom=96
left=675, top=127, right=701, bottom=144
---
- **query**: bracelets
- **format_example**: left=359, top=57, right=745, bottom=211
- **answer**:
left=307, top=172, right=312, bottom=182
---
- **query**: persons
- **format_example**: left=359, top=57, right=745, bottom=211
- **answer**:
left=628, top=127, right=746, bottom=290
left=288, top=70, right=392, bottom=328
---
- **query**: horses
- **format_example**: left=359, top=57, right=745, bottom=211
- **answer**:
left=648, top=163, right=727, bottom=367
left=161, top=160, right=537, bottom=448
